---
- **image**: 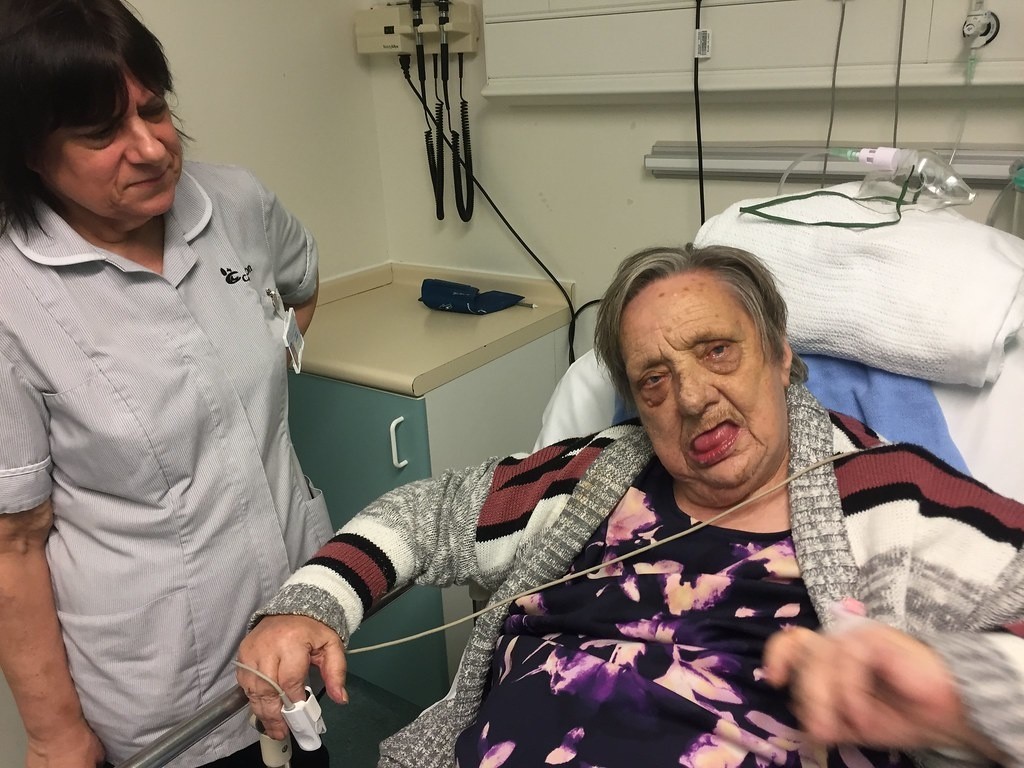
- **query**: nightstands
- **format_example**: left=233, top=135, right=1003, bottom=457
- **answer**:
left=289, top=280, right=574, bottom=708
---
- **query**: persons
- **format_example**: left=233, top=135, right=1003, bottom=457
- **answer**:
left=237, top=241, right=1024, bottom=768
left=0, top=1, right=335, bottom=768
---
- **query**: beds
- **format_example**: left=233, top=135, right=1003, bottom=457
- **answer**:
left=113, top=180, right=1024, bottom=768
